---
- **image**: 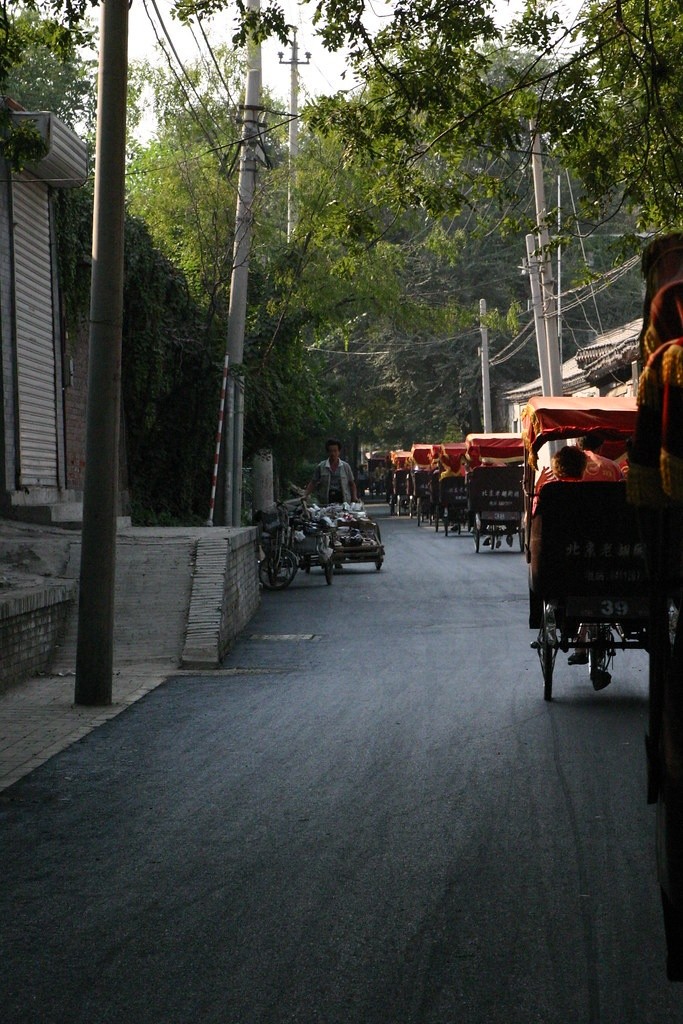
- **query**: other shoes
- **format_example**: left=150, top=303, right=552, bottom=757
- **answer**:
left=335, top=563, right=342, bottom=569
left=483, top=536, right=490, bottom=546
left=567, top=653, right=588, bottom=664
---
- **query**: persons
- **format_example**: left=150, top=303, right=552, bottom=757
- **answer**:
left=470, top=458, right=507, bottom=546
left=432, top=460, right=445, bottom=475
left=533, top=434, right=624, bottom=671
left=301, top=439, right=361, bottom=504
left=441, top=463, right=469, bottom=531
left=394, top=463, right=409, bottom=479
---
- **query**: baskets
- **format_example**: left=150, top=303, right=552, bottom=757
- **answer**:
left=260, top=502, right=280, bottom=531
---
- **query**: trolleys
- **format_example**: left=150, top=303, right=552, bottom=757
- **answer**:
left=301, top=494, right=386, bottom=575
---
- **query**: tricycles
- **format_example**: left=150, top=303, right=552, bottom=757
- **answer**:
left=462, top=434, right=528, bottom=554
left=365, top=442, right=472, bottom=538
left=634, top=231, right=682, bottom=981
left=521, top=392, right=683, bottom=701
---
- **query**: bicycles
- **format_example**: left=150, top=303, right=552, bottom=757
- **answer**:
left=254, top=496, right=307, bottom=591
